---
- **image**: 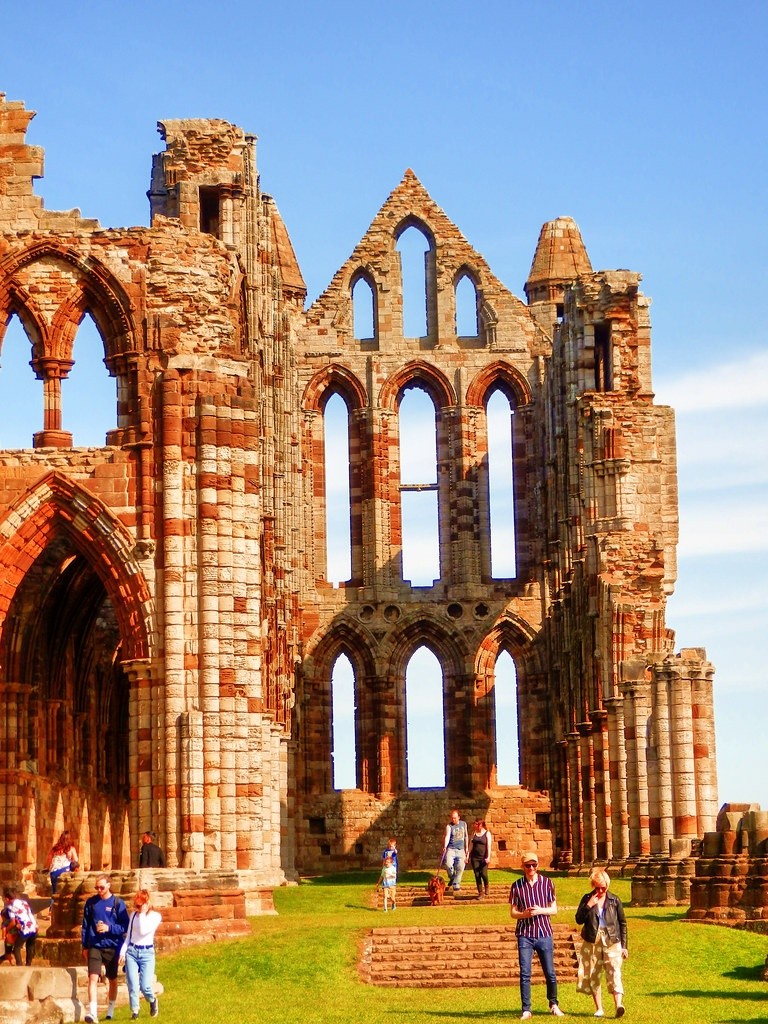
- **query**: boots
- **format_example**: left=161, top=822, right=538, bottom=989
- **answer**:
left=485, top=885, right=490, bottom=895
left=476, top=885, right=484, bottom=900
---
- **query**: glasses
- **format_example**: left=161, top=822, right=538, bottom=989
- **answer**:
left=525, top=863, right=537, bottom=868
left=94, top=886, right=104, bottom=890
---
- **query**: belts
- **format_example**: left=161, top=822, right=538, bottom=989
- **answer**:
left=130, top=943, right=153, bottom=949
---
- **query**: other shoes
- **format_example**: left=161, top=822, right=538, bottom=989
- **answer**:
left=106, top=1011, right=112, bottom=1019
left=150, top=997, right=158, bottom=1017
left=391, top=904, right=396, bottom=910
left=520, top=1011, right=532, bottom=1021
left=593, top=1010, right=604, bottom=1018
left=84, top=1014, right=98, bottom=1024
left=132, top=1012, right=138, bottom=1020
left=551, top=1004, right=564, bottom=1017
left=615, top=1006, right=625, bottom=1018
left=384, top=908, right=388, bottom=912
left=453, top=882, right=461, bottom=890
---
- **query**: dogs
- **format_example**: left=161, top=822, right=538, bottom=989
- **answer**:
left=427, top=875, right=446, bottom=906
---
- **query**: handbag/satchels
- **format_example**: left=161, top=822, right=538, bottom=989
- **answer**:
left=576, top=958, right=592, bottom=995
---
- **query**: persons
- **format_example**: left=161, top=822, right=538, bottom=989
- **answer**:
left=575, top=870, right=629, bottom=1019
left=80, top=874, right=130, bottom=1024
left=507, top=853, right=566, bottom=1022
left=4, top=887, right=39, bottom=966
left=69, top=861, right=80, bottom=871
left=42, top=829, right=79, bottom=920
left=138, top=831, right=165, bottom=868
left=0, top=908, right=21, bottom=966
left=375, top=857, right=396, bottom=912
left=443, top=810, right=469, bottom=891
left=382, top=839, right=399, bottom=882
left=465, top=817, right=492, bottom=900
left=116, top=889, right=159, bottom=1021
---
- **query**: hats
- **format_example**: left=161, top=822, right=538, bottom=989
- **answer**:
left=521, top=852, right=538, bottom=863
